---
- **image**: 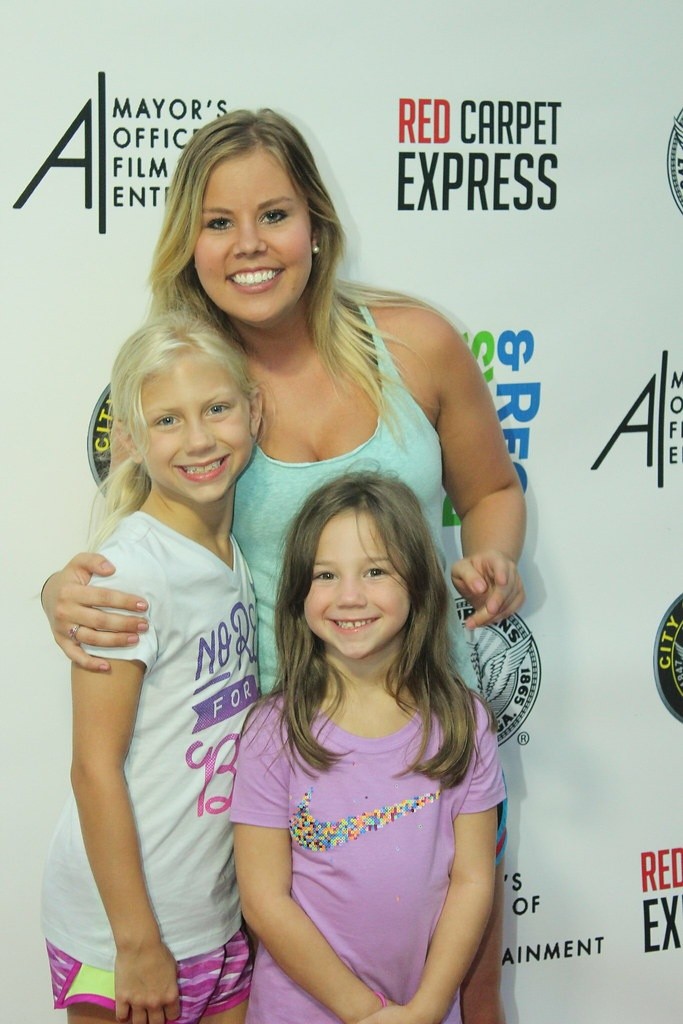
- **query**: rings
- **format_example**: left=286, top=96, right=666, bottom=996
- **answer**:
left=70, top=623, right=81, bottom=646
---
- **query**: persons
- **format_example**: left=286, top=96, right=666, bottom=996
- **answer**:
left=226, top=468, right=510, bottom=1024
left=36, top=102, right=530, bottom=1023
left=49, top=311, right=264, bottom=1023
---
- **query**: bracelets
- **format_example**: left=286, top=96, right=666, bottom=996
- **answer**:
left=373, top=990, right=389, bottom=1010
